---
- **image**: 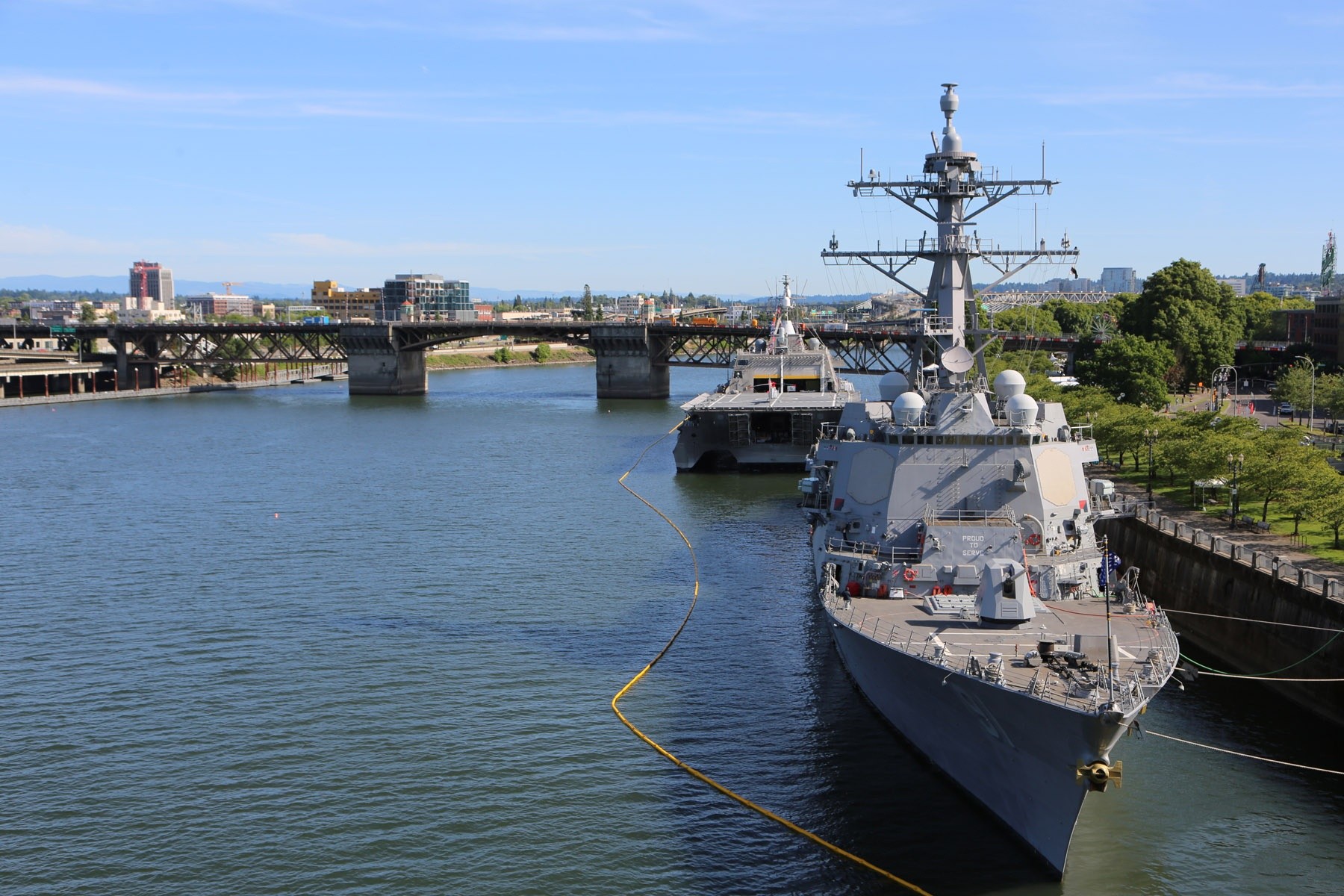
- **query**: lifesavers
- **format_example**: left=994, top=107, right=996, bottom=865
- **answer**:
left=932, top=585, right=952, bottom=596
left=903, top=568, right=915, bottom=582
left=1028, top=533, right=1040, bottom=545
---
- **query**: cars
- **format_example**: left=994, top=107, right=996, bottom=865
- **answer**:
left=1279, top=402, right=1292, bottom=416
left=1266, top=383, right=1277, bottom=394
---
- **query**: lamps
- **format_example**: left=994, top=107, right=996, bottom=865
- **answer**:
left=985, top=545, right=992, bottom=552
left=927, top=534, right=933, bottom=539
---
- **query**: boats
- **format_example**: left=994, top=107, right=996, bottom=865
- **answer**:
left=795, top=79, right=1182, bottom=884
left=672, top=273, right=870, bottom=475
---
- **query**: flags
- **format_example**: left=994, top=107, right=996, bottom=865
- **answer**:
left=1099, top=549, right=1121, bottom=587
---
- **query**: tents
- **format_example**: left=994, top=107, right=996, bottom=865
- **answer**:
left=1194, top=475, right=1234, bottom=512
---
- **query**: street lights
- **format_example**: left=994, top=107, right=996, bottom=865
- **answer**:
left=1220, top=365, right=1237, bottom=418
left=1226, top=451, right=1244, bottom=530
left=1143, top=429, right=1159, bottom=508
left=1294, top=355, right=1315, bottom=433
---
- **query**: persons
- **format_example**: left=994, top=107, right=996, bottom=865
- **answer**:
left=841, top=587, right=850, bottom=611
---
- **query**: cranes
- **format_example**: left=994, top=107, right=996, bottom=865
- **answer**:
left=131, top=262, right=162, bottom=297
